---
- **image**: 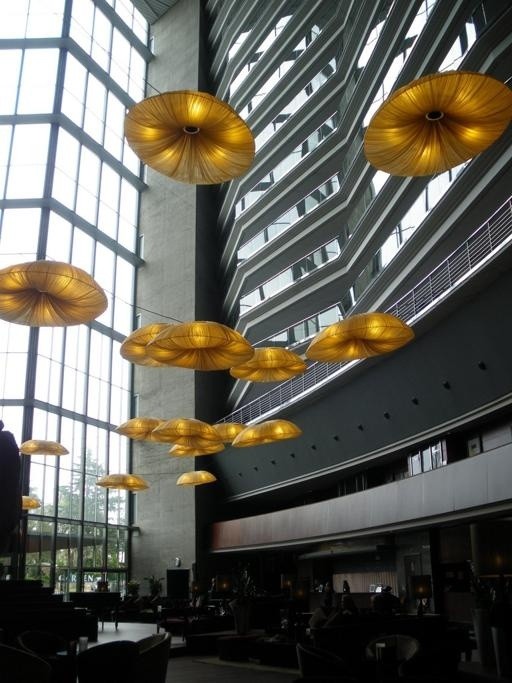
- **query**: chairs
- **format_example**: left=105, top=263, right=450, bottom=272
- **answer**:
left=1, top=628, right=172, bottom=682
left=293, top=632, right=420, bottom=682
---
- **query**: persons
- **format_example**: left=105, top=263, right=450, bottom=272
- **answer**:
left=319, top=581, right=338, bottom=618
left=327, top=593, right=359, bottom=625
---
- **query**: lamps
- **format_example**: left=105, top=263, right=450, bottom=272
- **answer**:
left=305, top=312, right=415, bottom=363
left=113, top=412, right=305, bottom=459
left=19, top=438, right=70, bottom=457
left=122, top=87, right=256, bottom=186
left=361, top=65, right=512, bottom=178
left=175, top=468, right=218, bottom=487
left=1, top=259, right=110, bottom=329
left=230, top=346, right=308, bottom=384
left=94, top=473, right=150, bottom=492
left=119, top=321, right=177, bottom=367
left=145, top=320, right=255, bottom=372
